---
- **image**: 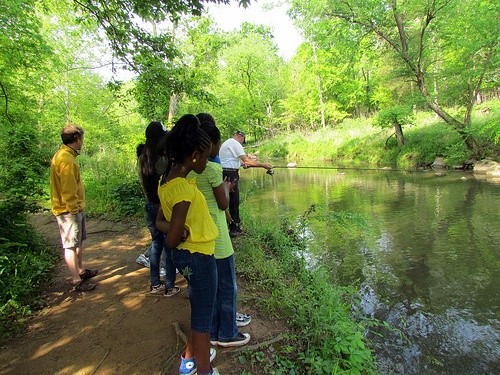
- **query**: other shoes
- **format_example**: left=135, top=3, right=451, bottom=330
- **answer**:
left=79, top=268, right=99, bottom=280
left=136, top=254, right=150, bottom=268
left=163, top=285, right=180, bottom=297
left=229, top=226, right=243, bottom=237
left=74, top=278, right=96, bottom=292
left=159, top=267, right=179, bottom=277
left=149, top=283, right=166, bottom=294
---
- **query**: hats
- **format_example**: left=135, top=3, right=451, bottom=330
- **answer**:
left=236, top=130, right=246, bottom=144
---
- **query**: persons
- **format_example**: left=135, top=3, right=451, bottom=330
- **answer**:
left=49, top=123, right=99, bottom=291
left=219, top=132, right=271, bottom=233
left=134, top=113, right=251, bottom=375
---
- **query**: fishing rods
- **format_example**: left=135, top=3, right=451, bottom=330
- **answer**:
left=273, top=165, right=405, bottom=173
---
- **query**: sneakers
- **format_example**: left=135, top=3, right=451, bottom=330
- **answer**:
left=178, top=347, right=221, bottom=375
left=235, top=311, right=251, bottom=327
left=209, top=330, right=251, bottom=348
